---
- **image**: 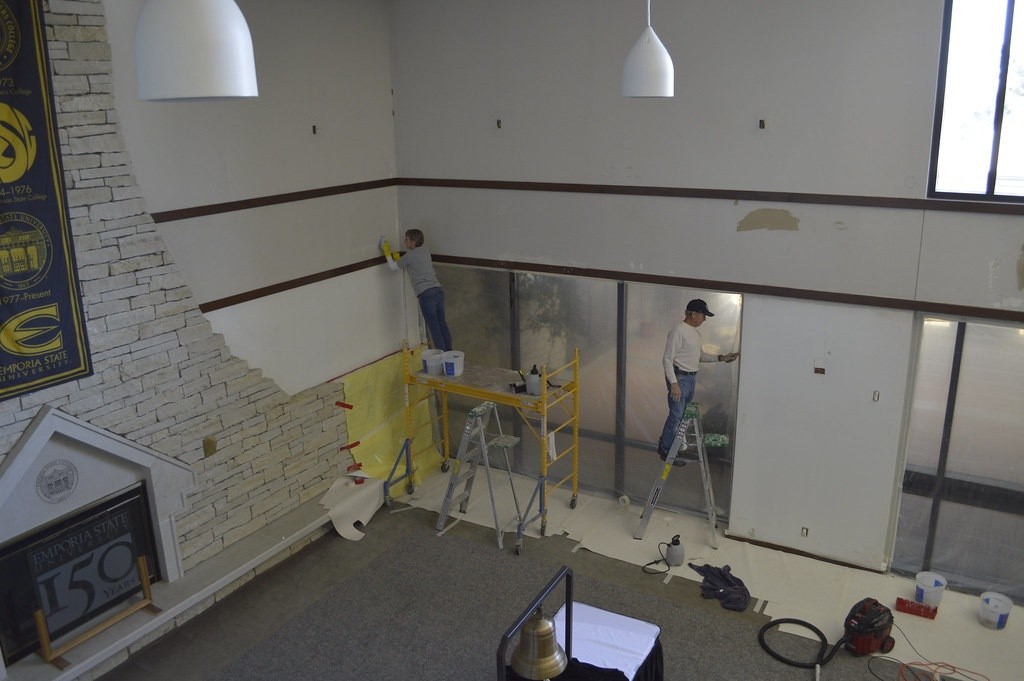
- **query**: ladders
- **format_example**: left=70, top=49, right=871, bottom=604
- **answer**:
left=631, top=401, right=720, bottom=550
left=433, top=401, right=522, bottom=551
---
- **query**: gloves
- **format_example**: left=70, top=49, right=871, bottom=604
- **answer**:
left=391, top=250, right=400, bottom=262
left=382, top=240, right=392, bottom=258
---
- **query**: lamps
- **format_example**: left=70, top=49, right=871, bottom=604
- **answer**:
left=135, top=0, right=258, bottom=101
left=621, top=0, right=676, bottom=96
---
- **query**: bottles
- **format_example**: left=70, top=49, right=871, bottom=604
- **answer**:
left=666, top=534, right=684, bottom=566
left=526, top=364, right=542, bottom=395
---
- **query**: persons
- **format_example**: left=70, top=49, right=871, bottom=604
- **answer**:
left=657, top=299, right=738, bottom=466
left=381, top=229, right=452, bottom=352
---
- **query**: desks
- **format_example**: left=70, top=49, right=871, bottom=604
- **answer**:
left=504, top=600, right=663, bottom=681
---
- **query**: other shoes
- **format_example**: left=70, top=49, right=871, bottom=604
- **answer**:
left=660, top=455, right=685, bottom=467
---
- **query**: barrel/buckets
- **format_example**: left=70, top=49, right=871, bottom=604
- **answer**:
left=442, top=350, right=464, bottom=377
left=915, top=571, right=947, bottom=606
left=421, top=348, right=445, bottom=375
left=978, top=591, right=1014, bottom=630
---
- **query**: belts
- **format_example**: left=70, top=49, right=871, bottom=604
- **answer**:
left=677, top=370, right=697, bottom=376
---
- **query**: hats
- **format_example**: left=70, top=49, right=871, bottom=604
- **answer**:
left=687, top=299, right=714, bottom=317
